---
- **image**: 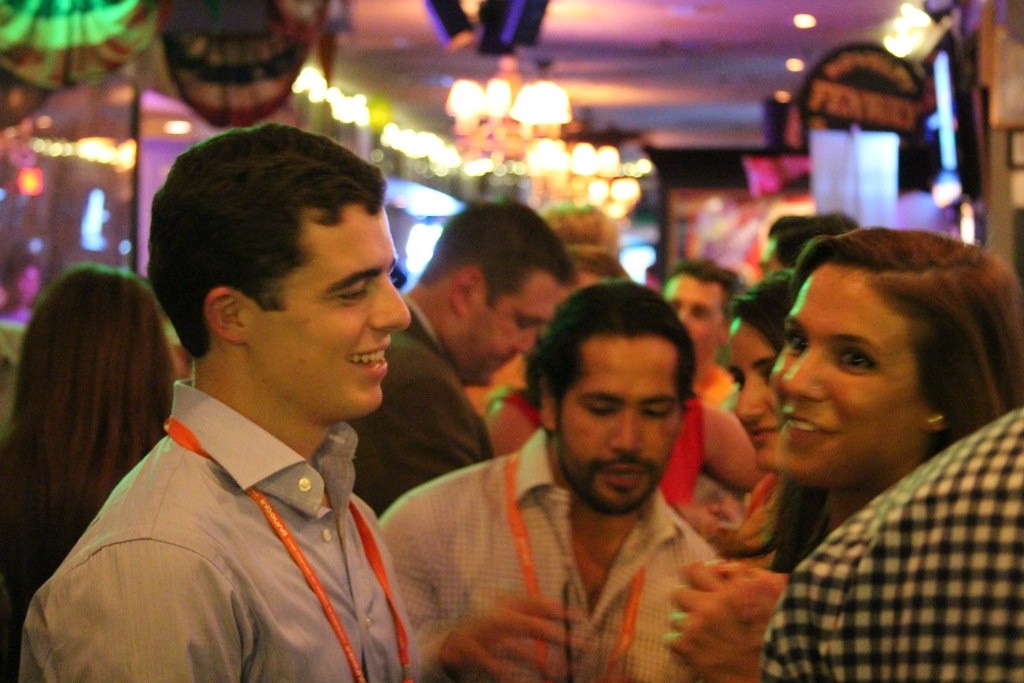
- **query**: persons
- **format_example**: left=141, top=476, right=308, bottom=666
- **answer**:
left=18, top=122, right=422, bottom=683
left=0, top=200, right=1024, bottom=683
left=377, top=281, right=718, bottom=683
